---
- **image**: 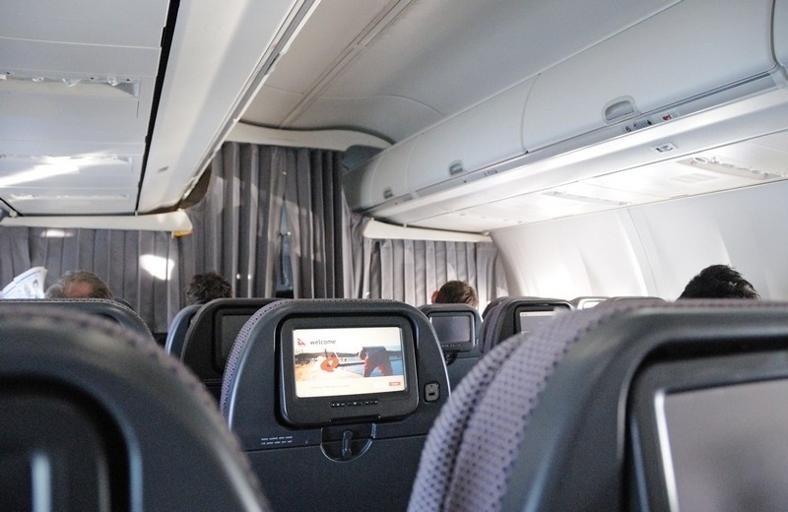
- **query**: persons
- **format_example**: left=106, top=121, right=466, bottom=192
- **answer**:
left=675, top=262, right=763, bottom=300
left=45, top=269, right=110, bottom=302
left=184, top=269, right=231, bottom=304
left=431, top=280, right=477, bottom=309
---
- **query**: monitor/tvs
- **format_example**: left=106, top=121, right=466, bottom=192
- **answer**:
left=428, top=311, right=476, bottom=352
left=515, top=303, right=571, bottom=333
left=213, top=308, right=259, bottom=369
left=280, top=316, right=420, bottom=426
left=629, top=349, right=788, bottom=512
